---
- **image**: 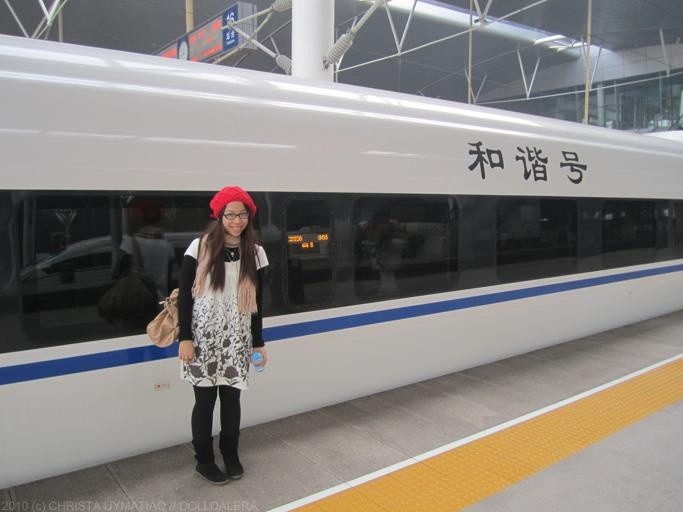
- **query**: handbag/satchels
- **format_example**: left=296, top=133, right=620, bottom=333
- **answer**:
left=98, top=267, right=158, bottom=319
left=146, top=288, right=180, bottom=348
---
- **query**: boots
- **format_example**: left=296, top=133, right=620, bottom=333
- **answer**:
left=192, top=437, right=229, bottom=484
left=219, top=433, right=243, bottom=479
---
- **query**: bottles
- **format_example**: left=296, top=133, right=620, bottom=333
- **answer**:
left=249, top=350, right=266, bottom=375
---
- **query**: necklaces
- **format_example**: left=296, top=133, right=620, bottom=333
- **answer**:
left=222, top=244, right=239, bottom=265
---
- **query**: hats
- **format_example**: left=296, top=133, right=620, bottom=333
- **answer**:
left=210, top=186, right=257, bottom=217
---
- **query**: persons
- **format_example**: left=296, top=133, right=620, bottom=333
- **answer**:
left=118, top=198, right=176, bottom=336
left=178, top=184, right=269, bottom=483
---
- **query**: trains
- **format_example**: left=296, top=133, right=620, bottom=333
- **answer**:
left=0, top=30, right=682, bottom=501
left=4, top=220, right=554, bottom=307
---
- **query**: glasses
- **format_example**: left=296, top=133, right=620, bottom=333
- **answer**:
left=224, top=213, right=249, bottom=220
